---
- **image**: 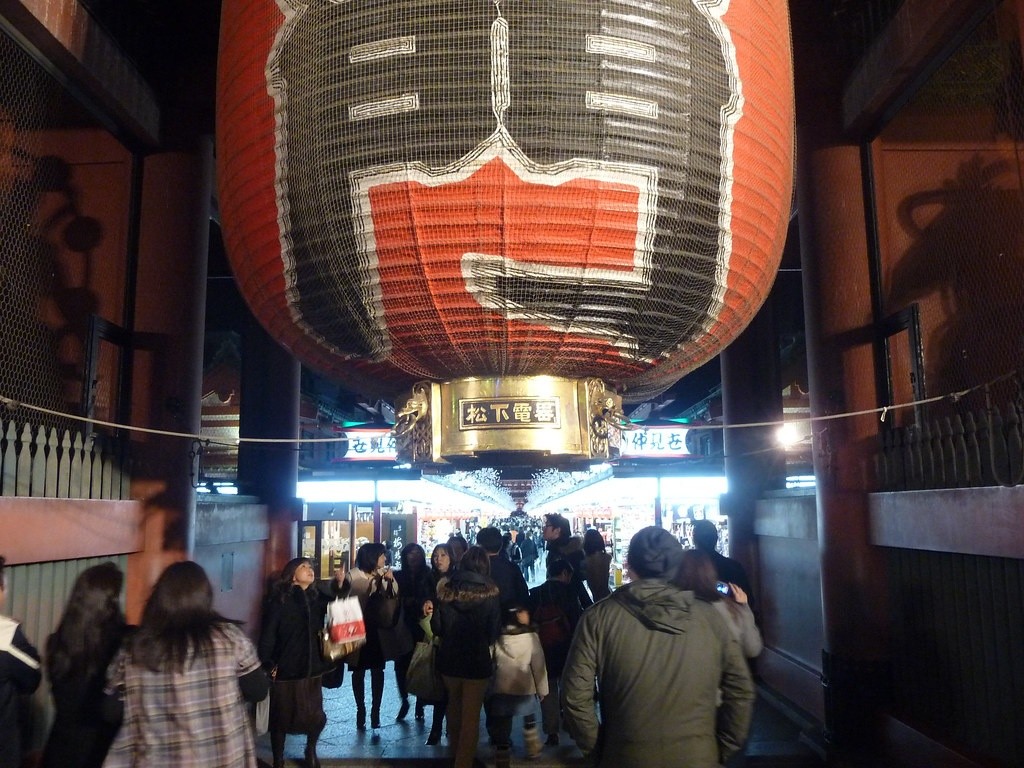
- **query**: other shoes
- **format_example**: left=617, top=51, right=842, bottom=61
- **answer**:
left=415, top=706, right=425, bottom=721
left=303, top=749, right=321, bottom=768
left=528, top=752, right=543, bottom=758
left=396, top=701, right=409, bottom=720
left=357, top=715, right=366, bottom=731
left=544, top=734, right=560, bottom=745
left=425, top=730, right=443, bottom=745
left=372, top=713, right=381, bottom=728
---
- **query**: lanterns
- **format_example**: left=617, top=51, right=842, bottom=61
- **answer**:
left=217, top=0, right=796, bottom=473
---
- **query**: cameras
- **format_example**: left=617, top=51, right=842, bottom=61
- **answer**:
left=716, top=581, right=731, bottom=595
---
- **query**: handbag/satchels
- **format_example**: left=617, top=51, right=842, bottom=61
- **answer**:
left=380, top=577, right=401, bottom=630
left=318, top=627, right=367, bottom=661
left=405, top=640, right=437, bottom=698
left=327, top=595, right=367, bottom=645
left=321, top=658, right=344, bottom=689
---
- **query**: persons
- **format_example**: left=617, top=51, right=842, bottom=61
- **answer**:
left=392, top=514, right=600, bottom=768
left=559, top=520, right=762, bottom=767
left=345, top=544, right=399, bottom=729
left=256, top=557, right=365, bottom=768
left=0, top=554, right=269, bottom=768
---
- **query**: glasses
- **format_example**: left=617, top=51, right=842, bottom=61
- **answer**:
left=542, top=523, right=553, bottom=528
